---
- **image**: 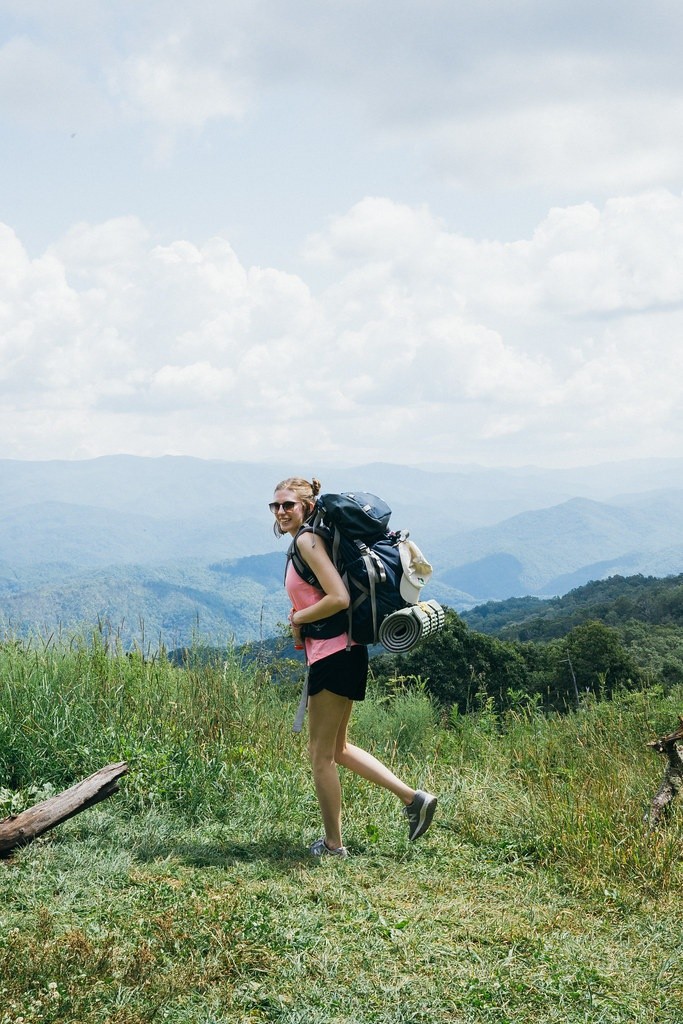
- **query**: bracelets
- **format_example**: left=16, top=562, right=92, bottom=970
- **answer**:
left=288, top=611, right=303, bottom=629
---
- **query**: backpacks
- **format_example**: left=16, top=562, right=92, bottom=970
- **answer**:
left=291, top=491, right=409, bottom=646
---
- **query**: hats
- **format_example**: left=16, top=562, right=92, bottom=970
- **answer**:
left=398, top=539, right=434, bottom=606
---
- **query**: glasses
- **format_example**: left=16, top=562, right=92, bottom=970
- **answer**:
left=269, top=501, right=304, bottom=514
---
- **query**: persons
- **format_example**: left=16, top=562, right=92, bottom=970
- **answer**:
left=269, top=478, right=438, bottom=857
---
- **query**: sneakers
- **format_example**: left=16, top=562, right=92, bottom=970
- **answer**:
left=402, top=790, right=438, bottom=841
left=308, top=838, right=348, bottom=862
